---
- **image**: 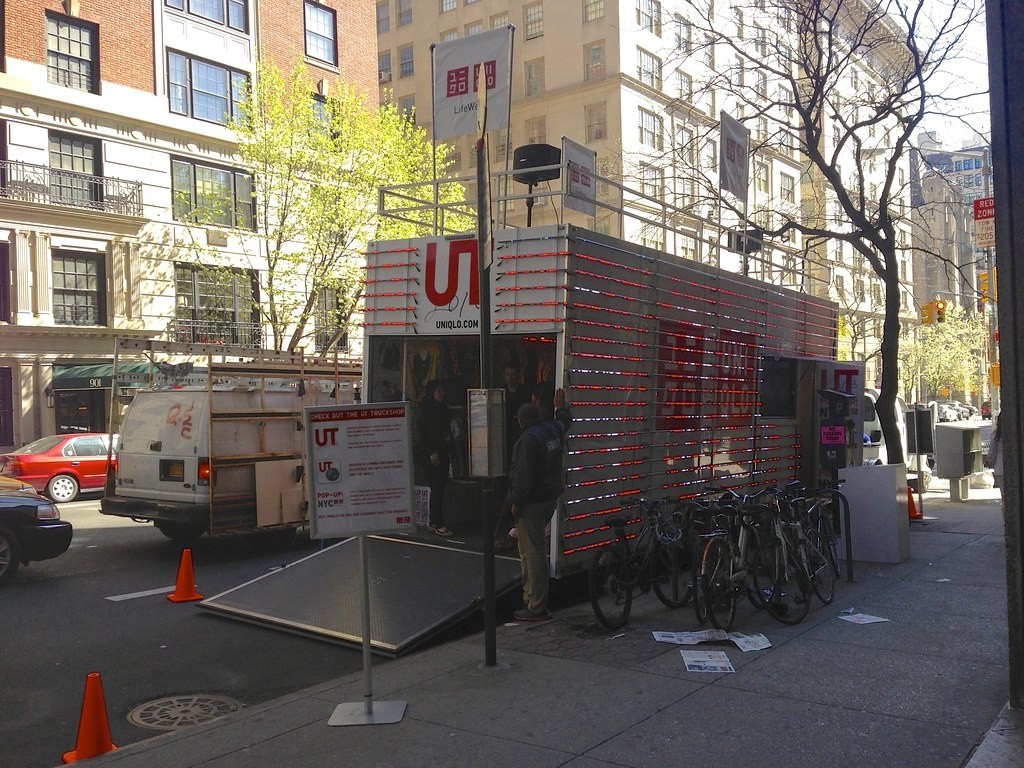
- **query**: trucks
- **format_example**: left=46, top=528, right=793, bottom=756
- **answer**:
left=197, top=220, right=884, bottom=664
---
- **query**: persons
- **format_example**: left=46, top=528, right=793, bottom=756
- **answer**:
left=982, top=410, right=1005, bottom=519
left=413, top=379, right=457, bottom=538
left=501, top=360, right=534, bottom=473
left=507, top=388, right=573, bottom=620
left=532, top=391, right=549, bottom=417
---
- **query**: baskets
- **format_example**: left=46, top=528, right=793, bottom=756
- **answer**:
left=642, top=505, right=677, bottom=523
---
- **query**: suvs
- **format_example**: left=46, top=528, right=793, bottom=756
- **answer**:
left=98, top=384, right=364, bottom=562
left=980, top=401, right=992, bottom=420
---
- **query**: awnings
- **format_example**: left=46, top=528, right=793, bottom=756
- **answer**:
left=51, top=361, right=172, bottom=388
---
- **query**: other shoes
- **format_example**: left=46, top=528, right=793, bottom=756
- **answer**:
left=436, top=526, right=454, bottom=537
left=428, top=525, right=437, bottom=531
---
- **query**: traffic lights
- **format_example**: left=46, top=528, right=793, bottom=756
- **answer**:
left=935, top=300, right=946, bottom=323
left=921, top=302, right=933, bottom=324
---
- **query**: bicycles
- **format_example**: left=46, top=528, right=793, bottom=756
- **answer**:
left=691, top=475, right=846, bottom=633
left=586, top=495, right=693, bottom=632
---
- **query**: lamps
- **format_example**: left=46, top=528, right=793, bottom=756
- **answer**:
left=44, top=388, right=55, bottom=408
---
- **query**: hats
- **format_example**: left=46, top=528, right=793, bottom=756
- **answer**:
left=514, top=404, right=540, bottom=419
left=534, top=382, right=555, bottom=406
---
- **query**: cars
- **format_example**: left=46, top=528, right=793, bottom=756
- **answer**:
left=0, top=431, right=120, bottom=504
left=938, top=399, right=978, bottom=423
left=0, top=485, right=75, bottom=584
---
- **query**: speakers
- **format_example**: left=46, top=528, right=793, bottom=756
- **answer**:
left=727, top=230, right=763, bottom=253
left=513, top=144, right=561, bottom=185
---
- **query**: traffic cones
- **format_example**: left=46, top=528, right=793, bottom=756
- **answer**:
left=165, top=546, right=204, bottom=603
left=905, top=484, right=924, bottom=520
left=60, top=671, right=119, bottom=763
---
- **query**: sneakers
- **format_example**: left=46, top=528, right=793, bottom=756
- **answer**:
left=513, top=609, right=552, bottom=620
